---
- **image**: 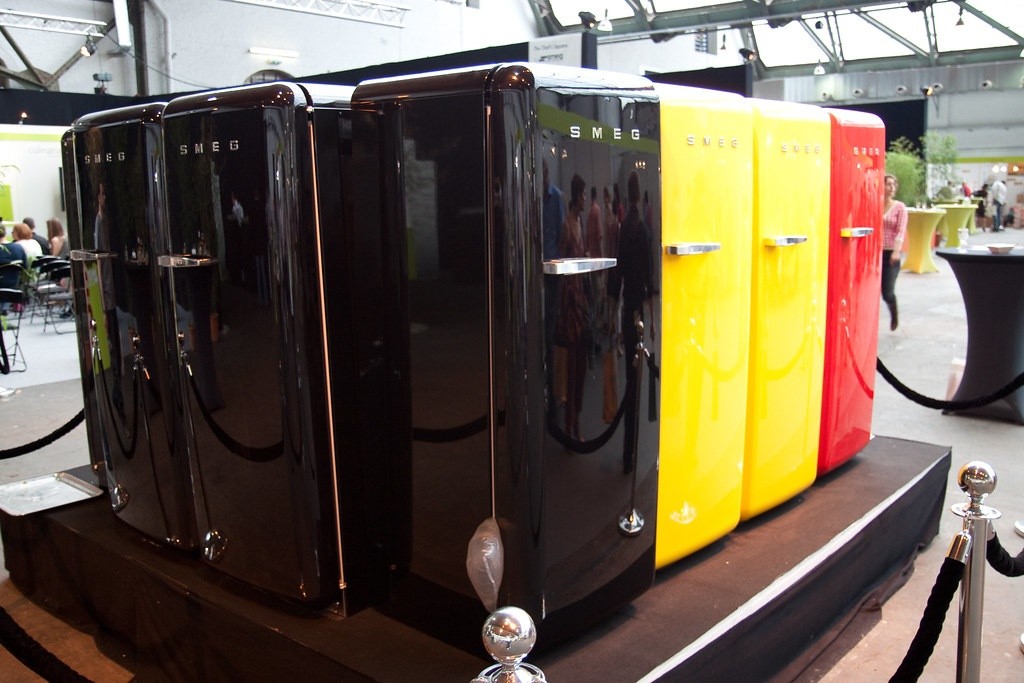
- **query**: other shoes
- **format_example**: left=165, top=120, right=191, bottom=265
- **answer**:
left=891, top=313, right=899, bottom=331
left=60, top=308, right=75, bottom=318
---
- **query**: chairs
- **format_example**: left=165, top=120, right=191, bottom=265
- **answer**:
left=24, top=254, right=74, bottom=334
left=0, top=260, right=27, bottom=373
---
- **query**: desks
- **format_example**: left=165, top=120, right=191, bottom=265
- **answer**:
left=899, top=206, right=946, bottom=276
left=934, top=246, right=1024, bottom=426
left=936, top=203, right=979, bottom=248
left=959, top=198, right=983, bottom=235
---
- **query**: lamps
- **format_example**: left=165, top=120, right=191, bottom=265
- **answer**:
left=921, top=85, right=933, bottom=98
left=738, top=48, right=756, bottom=65
left=577, top=11, right=613, bottom=33
left=956, top=7, right=964, bottom=25
left=80, top=33, right=97, bottom=58
left=813, top=59, right=825, bottom=75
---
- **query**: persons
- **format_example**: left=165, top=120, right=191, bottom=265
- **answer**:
left=960, top=182, right=972, bottom=196
left=12, top=224, right=42, bottom=273
left=881, top=175, right=909, bottom=330
left=24, top=218, right=50, bottom=254
left=990, top=180, right=1007, bottom=231
left=545, top=159, right=657, bottom=440
left=60, top=303, right=75, bottom=318
left=1004, top=208, right=1014, bottom=226
left=46, top=219, right=69, bottom=308
left=0, top=225, right=28, bottom=319
left=95, top=181, right=130, bottom=438
left=937, top=181, right=953, bottom=199
left=974, top=184, right=988, bottom=216
